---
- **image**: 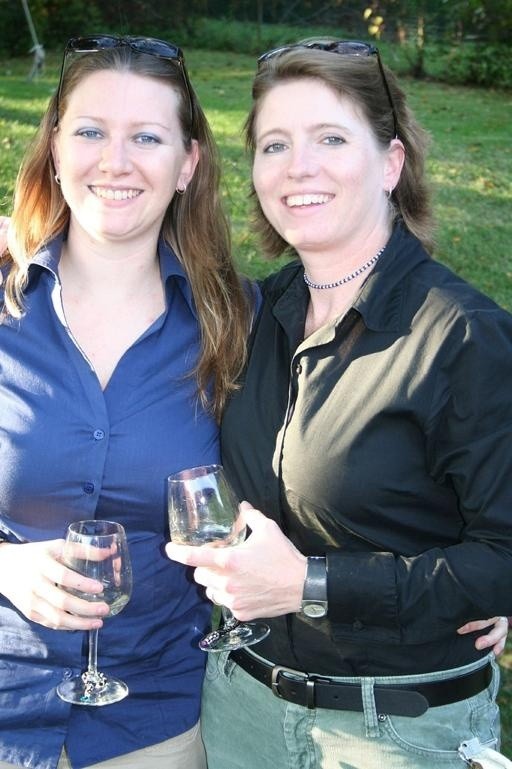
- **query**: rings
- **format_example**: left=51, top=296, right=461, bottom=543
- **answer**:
left=499, top=616, right=508, bottom=624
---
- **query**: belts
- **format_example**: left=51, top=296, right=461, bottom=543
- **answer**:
left=217, top=611, right=494, bottom=719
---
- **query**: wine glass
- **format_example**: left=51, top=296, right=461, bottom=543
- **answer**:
left=54, top=519, right=133, bottom=707
left=167, top=465, right=270, bottom=654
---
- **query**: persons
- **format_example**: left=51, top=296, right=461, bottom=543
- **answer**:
left=24, top=40, right=48, bottom=82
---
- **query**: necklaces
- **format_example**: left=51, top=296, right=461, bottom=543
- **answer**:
left=303, top=244, right=389, bottom=291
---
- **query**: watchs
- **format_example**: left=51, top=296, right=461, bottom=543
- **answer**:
left=301, top=555, right=330, bottom=623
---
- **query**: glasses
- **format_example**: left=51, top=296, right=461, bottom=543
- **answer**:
left=256, top=39, right=400, bottom=142
left=52, top=33, right=195, bottom=146
